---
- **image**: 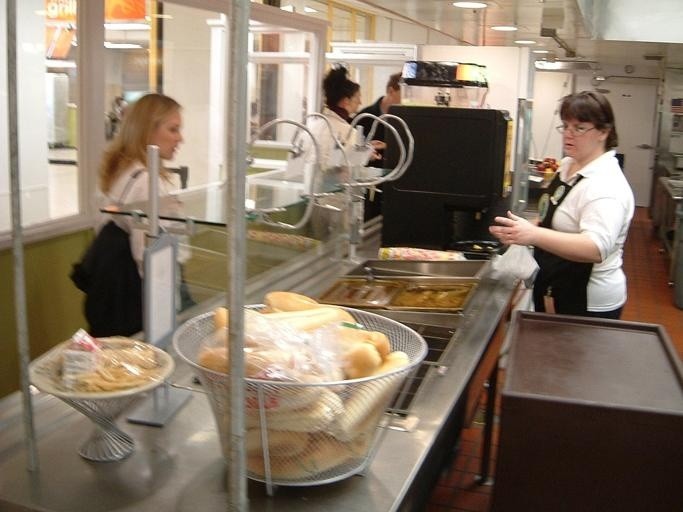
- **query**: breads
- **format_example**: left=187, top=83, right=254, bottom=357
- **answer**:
left=198, top=292, right=409, bottom=479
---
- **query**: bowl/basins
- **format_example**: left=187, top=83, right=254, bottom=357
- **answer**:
left=531, top=169, right=554, bottom=182
left=441, top=239, right=506, bottom=259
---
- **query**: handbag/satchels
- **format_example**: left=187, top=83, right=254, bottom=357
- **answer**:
left=69, top=168, right=146, bottom=338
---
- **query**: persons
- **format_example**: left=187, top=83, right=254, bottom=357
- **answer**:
left=485, top=89, right=636, bottom=323
left=69, top=92, right=198, bottom=337
left=304, top=64, right=384, bottom=201
left=351, top=69, right=406, bottom=219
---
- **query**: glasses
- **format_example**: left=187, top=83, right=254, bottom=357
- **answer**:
left=555, top=125, right=596, bottom=135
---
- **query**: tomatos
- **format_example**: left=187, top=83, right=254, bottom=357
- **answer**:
left=538, top=158, right=558, bottom=177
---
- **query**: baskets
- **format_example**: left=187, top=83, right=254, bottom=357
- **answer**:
left=172, top=302, right=429, bottom=488
left=28, top=336, right=174, bottom=462
